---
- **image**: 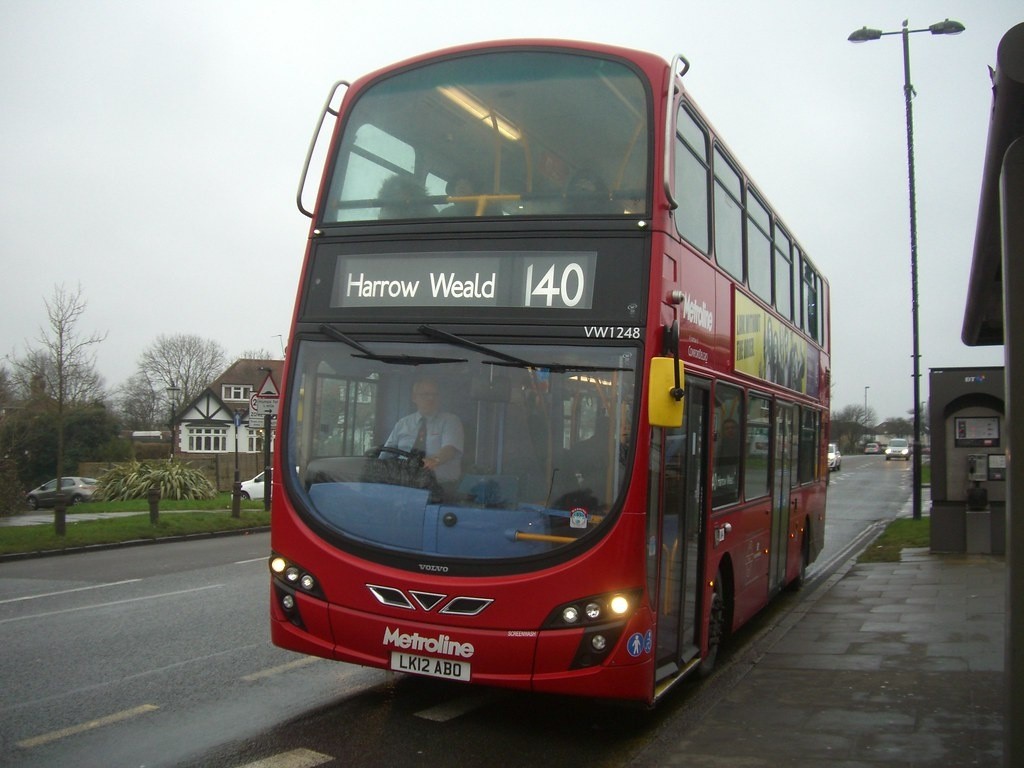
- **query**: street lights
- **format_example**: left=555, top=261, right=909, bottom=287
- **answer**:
left=166, top=380, right=181, bottom=463
left=863, top=386, right=870, bottom=442
left=846, top=19, right=966, bottom=520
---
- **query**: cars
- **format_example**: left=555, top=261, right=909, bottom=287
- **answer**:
left=863, top=442, right=881, bottom=455
left=827, top=444, right=842, bottom=472
left=26, top=477, right=102, bottom=512
left=885, top=438, right=909, bottom=461
left=230, top=466, right=301, bottom=503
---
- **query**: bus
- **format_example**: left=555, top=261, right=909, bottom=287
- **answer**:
left=267, top=38, right=830, bottom=708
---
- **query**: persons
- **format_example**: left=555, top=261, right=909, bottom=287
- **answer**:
left=719, top=418, right=741, bottom=466
left=763, top=317, right=806, bottom=393
left=571, top=416, right=629, bottom=502
left=377, top=376, right=465, bottom=503
left=438, top=169, right=504, bottom=217
left=377, top=175, right=439, bottom=219
left=531, top=152, right=612, bottom=201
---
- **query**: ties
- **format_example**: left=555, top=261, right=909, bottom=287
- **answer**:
left=408, top=422, right=427, bottom=458
